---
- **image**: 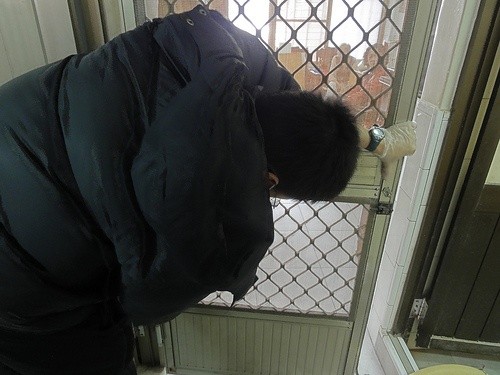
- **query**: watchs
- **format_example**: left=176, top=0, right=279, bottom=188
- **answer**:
left=365, top=124, right=385, bottom=152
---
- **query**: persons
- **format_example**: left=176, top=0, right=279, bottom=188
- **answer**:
left=345, top=43, right=396, bottom=127
left=0, top=3, right=416, bottom=375
left=322, top=43, right=359, bottom=101
